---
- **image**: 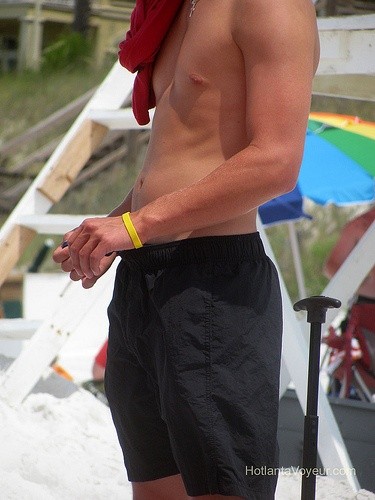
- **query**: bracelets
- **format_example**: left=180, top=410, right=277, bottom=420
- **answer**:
left=122, top=212, right=143, bottom=249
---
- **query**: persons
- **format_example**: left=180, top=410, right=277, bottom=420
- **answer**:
left=50, top=0, right=320, bottom=500
